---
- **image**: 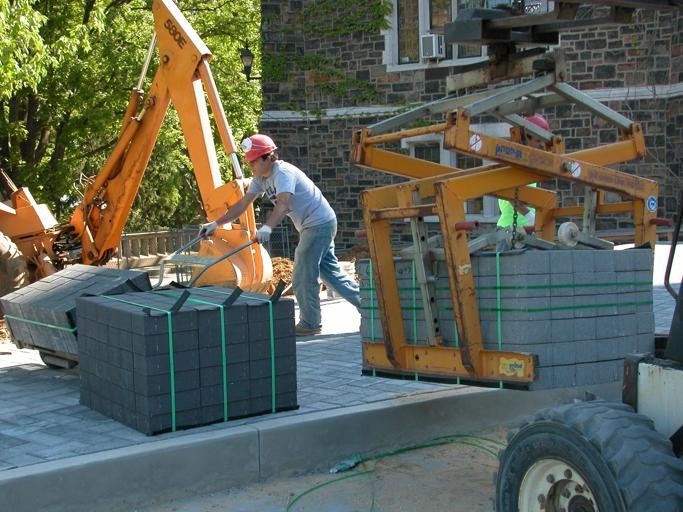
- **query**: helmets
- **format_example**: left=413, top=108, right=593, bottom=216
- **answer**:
left=243, top=133, right=279, bottom=162
left=522, top=115, right=550, bottom=133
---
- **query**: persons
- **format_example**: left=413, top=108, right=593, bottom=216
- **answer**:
left=199, top=133, right=360, bottom=338
left=495, top=114, right=551, bottom=253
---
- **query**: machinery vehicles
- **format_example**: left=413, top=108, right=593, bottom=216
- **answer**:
left=0, top=0, right=274, bottom=293
left=346, top=1, right=681, bottom=511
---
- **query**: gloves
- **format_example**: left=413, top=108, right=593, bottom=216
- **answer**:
left=524, top=210, right=536, bottom=226
left=256, top=224, right=272, bottom=243
left=197, top=221, right=218, bottom=239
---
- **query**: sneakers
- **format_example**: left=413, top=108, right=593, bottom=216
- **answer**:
left=295, top=323, right=323, bottom=336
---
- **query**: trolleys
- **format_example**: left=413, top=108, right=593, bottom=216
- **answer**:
left=9, top=231, right=256, bottom=365
